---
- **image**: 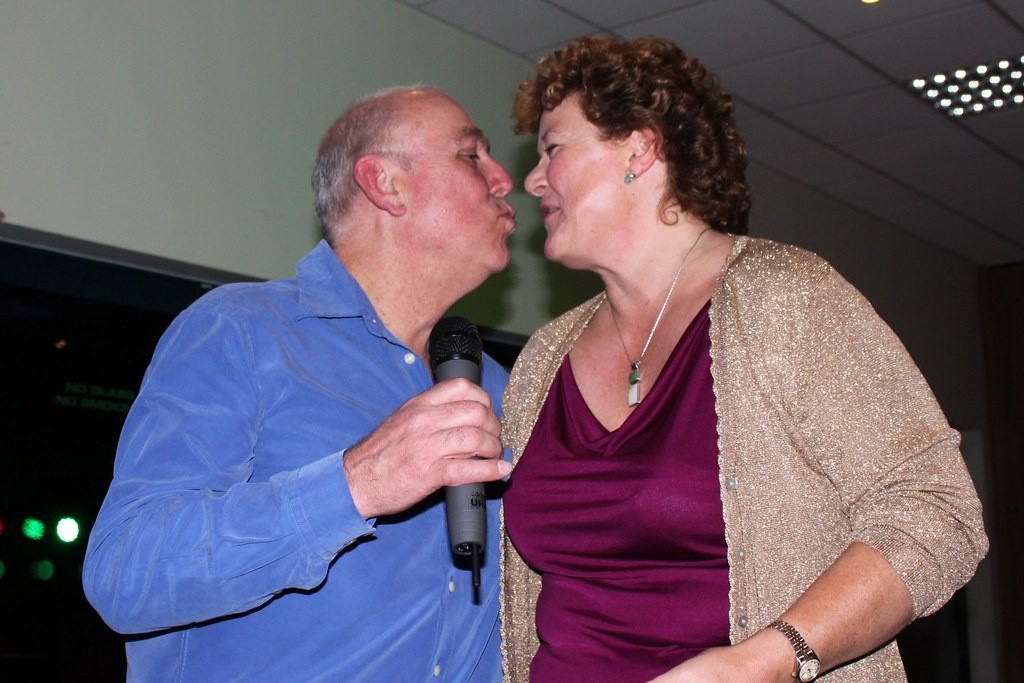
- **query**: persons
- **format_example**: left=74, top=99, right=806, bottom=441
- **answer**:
left=83, top=86, right=511, bottom=683
left=498, top=36, right=989, bottom=683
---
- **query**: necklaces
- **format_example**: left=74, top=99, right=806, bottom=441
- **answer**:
left=612, top=227, right=710, bottom=406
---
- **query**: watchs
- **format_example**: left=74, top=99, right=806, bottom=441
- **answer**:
left=768, top=622, right=821, bottom=683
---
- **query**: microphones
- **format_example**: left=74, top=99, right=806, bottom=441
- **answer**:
left=428, top=317, right=488, bottom=588
